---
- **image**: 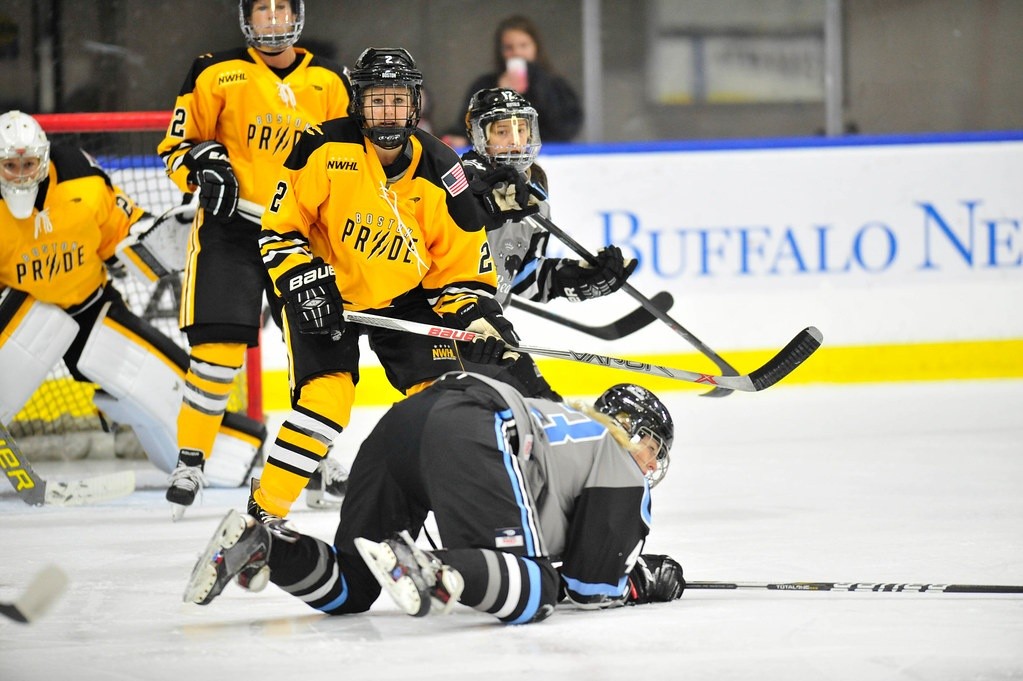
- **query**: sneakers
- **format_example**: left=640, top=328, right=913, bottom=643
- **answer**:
left=166, top=448, right=211, bottom=522
left=234, top=476, right=286, bottom=592
left=305, top=457, right=352, bottom=509
left=184, top=509, right=297, bottom=606
left=354, top=529, right=465, bottom=617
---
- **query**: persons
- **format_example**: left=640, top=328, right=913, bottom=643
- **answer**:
left=0, top=110, right=350, bottom=509
left=454, top=88, right=637, bottom=401
left=235, top=49, right=519, bottom=592
left=446, top=17, right=585, bottom=145
left=181, top=372, right=684, bottom=626
left=156, top=0, right=349, bottom=521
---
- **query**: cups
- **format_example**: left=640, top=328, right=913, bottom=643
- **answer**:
left=506, top=57, right=528, bottom=93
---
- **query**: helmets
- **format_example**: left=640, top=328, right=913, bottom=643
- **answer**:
left=0, top=110, right=52, bottom=219
left=346, top=46, right=425, bottom=150
left=239, top=1, right=305, bottom=55
left=465, top=86, right=541, bottom=173
left=595, top=384, right=675, bottom=488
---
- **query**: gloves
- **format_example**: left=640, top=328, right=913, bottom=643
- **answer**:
left=277, top=254, right=345, bottom=342
left=553, top=245, right=637, bottom=303
left=472, top=165, right=539, bottom=222
left=183, top=139, right=238, bottom=219
left=627, top=553, right=687, bottom=606
left=446, top=294, right=520, bottom=366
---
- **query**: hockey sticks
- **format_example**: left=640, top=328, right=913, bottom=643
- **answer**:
left=0, top=565, right=72, bottom=625
left=0, top=420, right=137, bottom=510
left=680, top=581, right=1023, bottom=595
left=504, top=288, right=674, bottom=342
left=340, top=304, right=826, bottom=394
left=528, top=208, right=743, bottom=399
